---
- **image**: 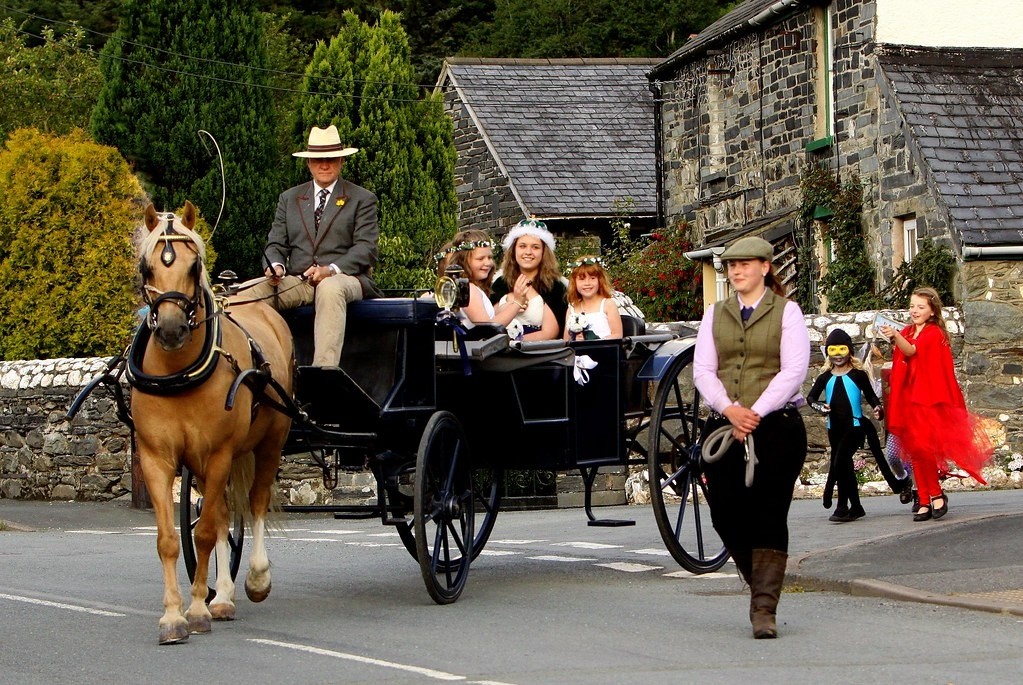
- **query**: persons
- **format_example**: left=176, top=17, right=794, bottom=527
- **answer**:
left=879, top=287, right=1006, bottom=524
left=805, top=328, right=883, bottom=523
left=421, top=216, right=624, bottom=342
left=236, top=125, right=385, bottom=370
left=691, top=236, right=810, bottom=639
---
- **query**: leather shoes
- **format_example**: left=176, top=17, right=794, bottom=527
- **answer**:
left=913, top=504, right=932, bottom=522
left=931, top=494, right=948, bottom=519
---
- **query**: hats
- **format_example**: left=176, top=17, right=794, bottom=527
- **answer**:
left=825, top=328, right=855, bottom=357
left=291, top=125, right=359, bottom=158
left=721, top=236, right=775, bottom=262
left=500, top=217, right=557, bottom=252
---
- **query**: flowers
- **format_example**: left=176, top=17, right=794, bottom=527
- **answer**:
left=567, top=257, right=611, bottom=272
left=433, top=238, right=497, bottom=260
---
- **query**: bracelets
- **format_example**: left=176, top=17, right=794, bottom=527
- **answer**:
left=328, top=265, right=336, bottom=276
left=514, top=300, right=523, bottom=308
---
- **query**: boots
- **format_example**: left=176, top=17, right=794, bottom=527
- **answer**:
left=749, top=548, right=788, bottom=639
left=911, top=488, right=922, bottom=512
left=895, top=469, right=913, bottom=504
left=727, top=546, right=751, bottom=586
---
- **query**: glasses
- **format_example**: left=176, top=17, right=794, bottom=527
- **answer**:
left=826, top=344, right=850, bottom=358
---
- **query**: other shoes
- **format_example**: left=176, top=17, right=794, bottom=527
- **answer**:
left=841, top=504, right=866, bottom=521
left=829, top=505, right=849, bottom=521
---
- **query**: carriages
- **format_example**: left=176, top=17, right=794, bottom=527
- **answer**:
left=64, top=199, right=732, bottom=645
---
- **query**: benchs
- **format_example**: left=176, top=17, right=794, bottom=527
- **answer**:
left=621, top=316, right=680, bottom=366
left=281, top=288, right=444, bottom=329
left=434, top=318, right=574, bottom=370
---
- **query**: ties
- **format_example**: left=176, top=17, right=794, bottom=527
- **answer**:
left=314, top=189, right=330, bottom=236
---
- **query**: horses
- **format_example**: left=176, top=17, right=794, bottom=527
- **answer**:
left=131, top=198, right=293, bottom=646
